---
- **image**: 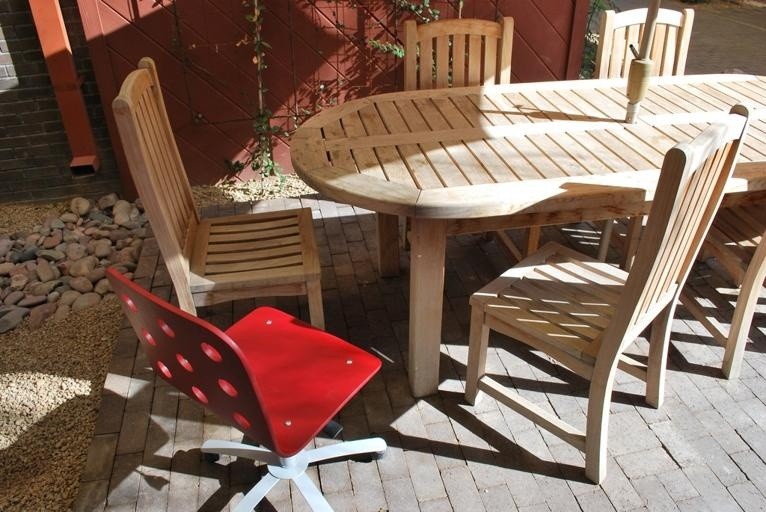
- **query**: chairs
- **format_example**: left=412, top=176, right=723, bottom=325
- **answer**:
left=112, top=57, right=326, bottom=332
left=594, top=8, right=695, bottom=271
left=106, top=267, right=388, bottom=512
left=464, top=102, right=751, bottom=484
left=679, top=206, right=766, bottom=381
left=403, top=16, right=540, bottom=261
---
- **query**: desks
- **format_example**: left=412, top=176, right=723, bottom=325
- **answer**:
left=290, top=74, right=765, bottom=397
